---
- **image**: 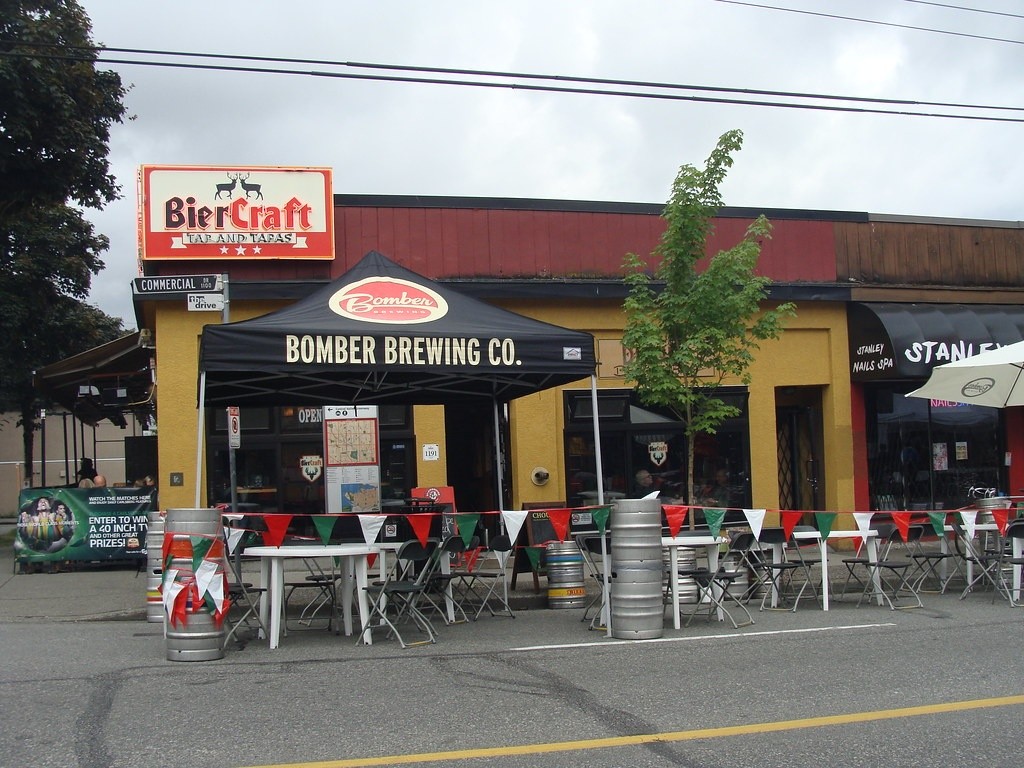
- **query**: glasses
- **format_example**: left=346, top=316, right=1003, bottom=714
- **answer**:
left=642, top=475, right=652, bottom=479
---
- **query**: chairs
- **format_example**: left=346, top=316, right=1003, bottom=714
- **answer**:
left=225, top=519, right=1024, bottom=650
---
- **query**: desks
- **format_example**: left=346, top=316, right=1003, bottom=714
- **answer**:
left=244, top=545, right=380, bottom=649
left=341, top=543, right=455, bottom=626
left=661, top=536, right=724, bottom=629
left=939, top=523, right=1022, bottom=602
left=771, top=530, right=884, bottom=608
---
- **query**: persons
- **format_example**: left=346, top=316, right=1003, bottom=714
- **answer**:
left=21, top=498, right=71, bottom=542
left=697, top=471, right=730, bottom=508
left=634, top=470, right=663, bottom=498
left=77, top=458, right=98, bottom=482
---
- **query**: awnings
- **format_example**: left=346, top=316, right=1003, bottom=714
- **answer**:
left=846, top=300, right=1024, bottom=380
left=31, top=330, right=151, bottom=428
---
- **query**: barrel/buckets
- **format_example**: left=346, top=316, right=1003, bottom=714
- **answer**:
left=610, top=498, right=664, bottom=639
left=161, top=508, right=226, bottom=662
left=718, top=552, right=748, bottom=598
left=662, top=546, right=698, bottom=604
left=146, top=511, right=165, bottom=623
left=545, top=541, right=585, bottom=608
left=977, top=497, right=1014, bottom=584
left=752, top=549, right=783, bottom=599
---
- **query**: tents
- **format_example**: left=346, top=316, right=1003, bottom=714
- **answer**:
left=197, top=248, right=614, bottom=639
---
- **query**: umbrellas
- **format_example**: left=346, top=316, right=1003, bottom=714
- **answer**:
left=904, top=338, right=1023, bottom=408
